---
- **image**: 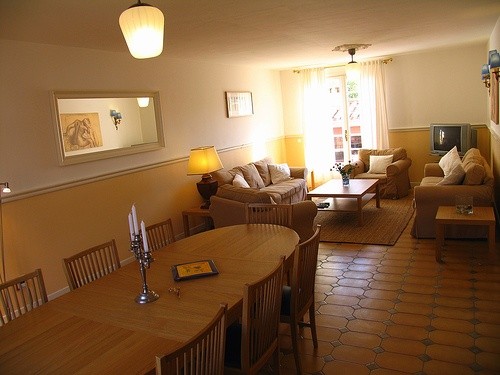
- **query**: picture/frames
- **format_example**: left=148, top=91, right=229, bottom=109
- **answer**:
left=225, top=90, right=255, bottom=118
left=59, top=112, right=104, bottom=152
left=171, top=258, right=220, bottom=284
left=489, top=67, right=499, bottom=124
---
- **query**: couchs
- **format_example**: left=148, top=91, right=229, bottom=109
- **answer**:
left=214, top=162, right=309, bottom=210
left=411, top=148, right=495, bottom=240
left=340, top=147, right=412, bottom=199
left=208, top=196, right=318, bottom=229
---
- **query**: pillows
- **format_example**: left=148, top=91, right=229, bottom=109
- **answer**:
left=437, top=146, right=462, bottom=176
left=254, top=150, right=278, bottom=188
left=367, top=154, right=394, bottom=174
left=441, top=162, right=467, bottom=185
left=211, top=168, right=244, bottom=186
left=233, top=172, right=251, bottom=189
left=268, top=162, right=294, bottom=185
left=239, top=163, right=265, bottom=189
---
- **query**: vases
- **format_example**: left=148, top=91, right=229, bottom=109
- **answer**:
left=341, top=175, right=350, bottom=187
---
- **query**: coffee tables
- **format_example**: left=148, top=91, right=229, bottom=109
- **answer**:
left=306, top=177, right=382, bottom=227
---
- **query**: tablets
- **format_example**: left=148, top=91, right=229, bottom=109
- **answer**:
left=172, top=258, right=219, bottom=281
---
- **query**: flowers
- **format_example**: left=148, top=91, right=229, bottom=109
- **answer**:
left=330, top=160, right=359, bottom=179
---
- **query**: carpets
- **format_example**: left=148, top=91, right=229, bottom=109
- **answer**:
left=301, top=191, right=415, bottom=245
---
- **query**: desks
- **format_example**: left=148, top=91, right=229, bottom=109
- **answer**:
left=434, top=205, right=496, bottom=263
left=182, top=202, right=213, bottom=237
left=1, top=220, right=302, bottom=374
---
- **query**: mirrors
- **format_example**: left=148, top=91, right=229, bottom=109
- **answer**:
left=49, top=86, right=167, bottom=167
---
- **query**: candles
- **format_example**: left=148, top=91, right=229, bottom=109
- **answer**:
left=131, top=202, right=141, bottom=241
left=127, top=213, right=135, bottom=244
left=140, top=219, right=150, bottom=254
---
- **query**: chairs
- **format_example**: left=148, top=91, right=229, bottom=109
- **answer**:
left=245, top=201, right=294, bottom=286
left=62, top=239, right=123, bottom=294
left=128, top=219, right=178, bottom=262
left=222, top=254, right=285, bottom=375
left=156, top=303, right=228, bottom=375
left=272, top=224, right=321, bottom=371
left=1, top=269, right=49, bottom=329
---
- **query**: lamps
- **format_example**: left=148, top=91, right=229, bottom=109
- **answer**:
left=0, top=182, right=12, bottom=195
left=481, top=63, right=491, bottom=89
left=185, top=145, right=225, bottom=210
left=488, top=49, right=497, bottom=66
left=136, top=98, right=151, bottom=108
left=110, top=109, right=122, bottom=130
left=118, top=0, right=164, bottom=61
left=331, top=42, right=371, bottom=84
left=489, top=53, right=499, bottom=83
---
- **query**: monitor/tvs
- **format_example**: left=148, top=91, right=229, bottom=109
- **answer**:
left=431, top=123, right=477, bottom=155
